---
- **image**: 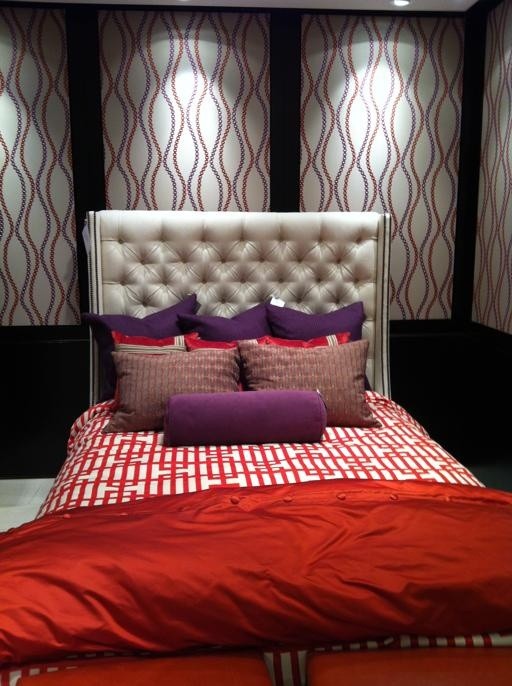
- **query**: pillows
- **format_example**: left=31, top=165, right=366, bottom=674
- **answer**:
left=79, top=293, right=381, bottom=447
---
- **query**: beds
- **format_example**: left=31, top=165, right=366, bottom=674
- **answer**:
left=0, top=207, right=510, bottom=686
